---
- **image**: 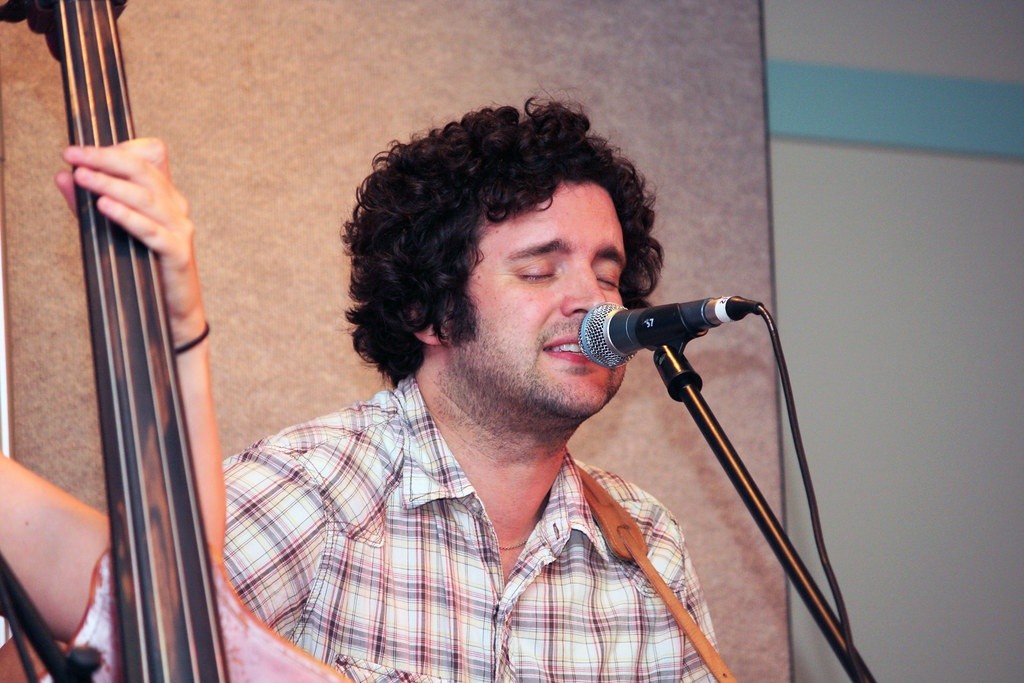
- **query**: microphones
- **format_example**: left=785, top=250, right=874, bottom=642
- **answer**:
left=578, top=295, right=765, bottom=369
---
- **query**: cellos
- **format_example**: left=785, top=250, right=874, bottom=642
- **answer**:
left=1, top=0, right=352, bottom=683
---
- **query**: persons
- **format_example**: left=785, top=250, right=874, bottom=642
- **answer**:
left=223, top=102, right=716, bottom=683
left=0, top=136, right=222, bottom=682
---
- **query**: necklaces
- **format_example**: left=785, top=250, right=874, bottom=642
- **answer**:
left=499, top=540, right=527, bottom=550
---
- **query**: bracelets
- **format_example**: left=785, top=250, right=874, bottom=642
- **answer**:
left=174, top=322, right=209, bottom=354
